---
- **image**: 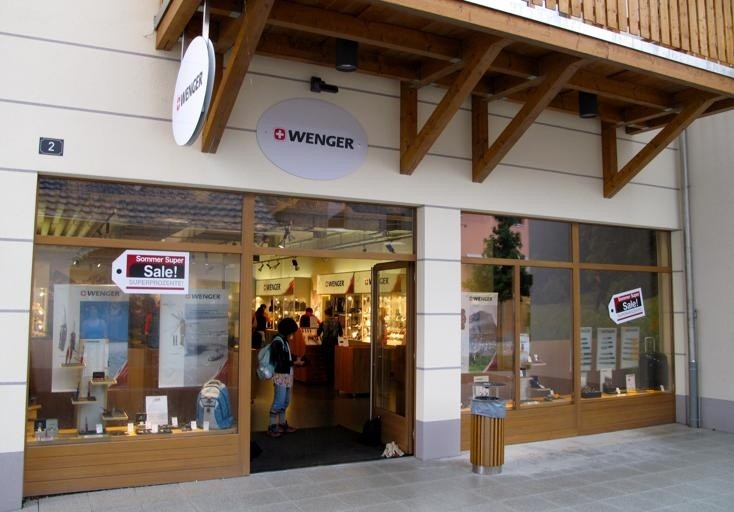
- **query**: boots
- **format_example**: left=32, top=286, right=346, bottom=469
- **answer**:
left=268, top=424, right=281, bottom=437
left=278, top=423, right=296, bottom=433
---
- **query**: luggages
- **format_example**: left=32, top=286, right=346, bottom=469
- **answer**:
left=639, top=337, right=669, bottom=388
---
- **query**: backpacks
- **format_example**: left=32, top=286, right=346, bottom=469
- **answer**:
left=196, top=381, right=234, bottom=430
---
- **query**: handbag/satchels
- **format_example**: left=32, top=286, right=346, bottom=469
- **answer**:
left=257, top=337, right=284, bottom=380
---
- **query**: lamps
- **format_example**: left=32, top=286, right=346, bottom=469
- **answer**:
left=274, top=227, right=290, bottom=250
left=254, top=257, right=302, bottom=272
left=334, top=32, right=359, bottom=74
left=577, top=85, right=599, bottom=122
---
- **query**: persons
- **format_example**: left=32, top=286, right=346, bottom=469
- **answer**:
left=81, top=303, right=109, bottom=339
left=298, top=307, right=321, bottom=327
left=377, top=305, right=388, bottom=385
left=315, top=307, right=345, bottom=389
left=268, top=316, right=300, bottom=437
left=255, top=304, right=270, bottom=342
left=141, top=297, right=160, bottom=345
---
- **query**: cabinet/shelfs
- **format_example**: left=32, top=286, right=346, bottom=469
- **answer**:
left=262, top=294, right=404, bottom=397
left=62, top=336, right=126, bottom=436
left=518, top=329, right=553, bottom=402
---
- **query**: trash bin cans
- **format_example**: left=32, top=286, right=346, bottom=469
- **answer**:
left=471, top=382, right=505, bottom=475
